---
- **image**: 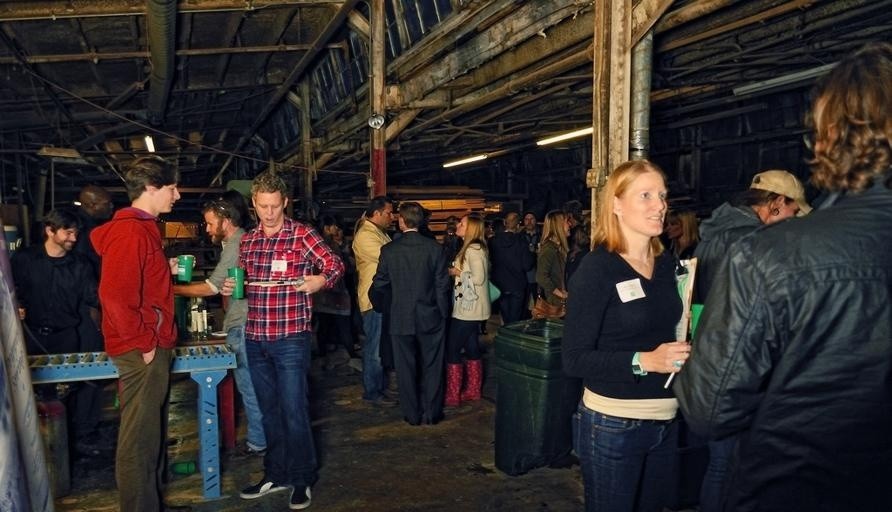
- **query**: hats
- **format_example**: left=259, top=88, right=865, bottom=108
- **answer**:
left=749, top=169, right=813, bottom=216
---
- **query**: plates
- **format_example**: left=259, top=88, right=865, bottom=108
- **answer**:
left=250, top=282, right=298, bottom=286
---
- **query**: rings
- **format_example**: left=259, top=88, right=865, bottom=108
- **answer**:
left=675, top=360, right=682, bottom=367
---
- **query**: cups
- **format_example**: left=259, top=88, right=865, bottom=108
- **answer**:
left=228, top=267, right=245, bottom=302
left=690, top=303, right=704, bottom=341
left=178, top=255, right=195, bottom=282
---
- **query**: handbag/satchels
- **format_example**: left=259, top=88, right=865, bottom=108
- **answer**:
left=486, top=280, right=501, bottom=304
left=531, top=295, right=568, bottom=320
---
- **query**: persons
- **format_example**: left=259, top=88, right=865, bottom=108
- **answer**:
left=221, top=189, right=258, bottom=232
left=173, top=194, right=268, bottom=463
left=689, top=169, right=813, bottom=306
left=9, top=207, right=101, bottom=466
left=87, top=153, right=183, bottom=512
left=218, top=170, right=346, bottom=509
left=674, top=37, right=892, bottom=512
left=561, top=160, right=694, bottom=511
left=69, top=184, right=114, bottom=263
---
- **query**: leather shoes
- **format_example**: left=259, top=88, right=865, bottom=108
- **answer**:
left=363, top=391, right=400, bottom=406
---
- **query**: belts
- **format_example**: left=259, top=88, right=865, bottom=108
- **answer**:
left=30, top=325, right=69, bottom=337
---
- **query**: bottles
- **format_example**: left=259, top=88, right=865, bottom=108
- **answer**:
left=174, top=295, right=229, bottom=340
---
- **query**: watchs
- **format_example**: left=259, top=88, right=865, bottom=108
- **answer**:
left=632, top=351, right=649, bottom=378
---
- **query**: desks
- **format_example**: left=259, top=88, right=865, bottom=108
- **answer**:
left=167, top=305, right=236, bottom=450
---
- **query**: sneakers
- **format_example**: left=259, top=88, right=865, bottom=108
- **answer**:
left=403, top=411, right=422, bottom=427
left=426, top=410, right=446, bottom=426
left=288, top=479, right=313, bottom=510
left=239, top=473, right=290, bottom=501
left=159, top=504, right=194, bottom=512
left=230, top=440, right=271, bottom=461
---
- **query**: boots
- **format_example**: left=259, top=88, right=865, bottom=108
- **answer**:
left=440, top=363, right=463, bottom=408
left=460, top=359, right=483, bottom=402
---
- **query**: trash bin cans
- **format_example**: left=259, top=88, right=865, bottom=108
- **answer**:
left=494, top=317, right=584, bottom=476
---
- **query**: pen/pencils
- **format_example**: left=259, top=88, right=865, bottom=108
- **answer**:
left=664, top=340, right=692, bottom=389
